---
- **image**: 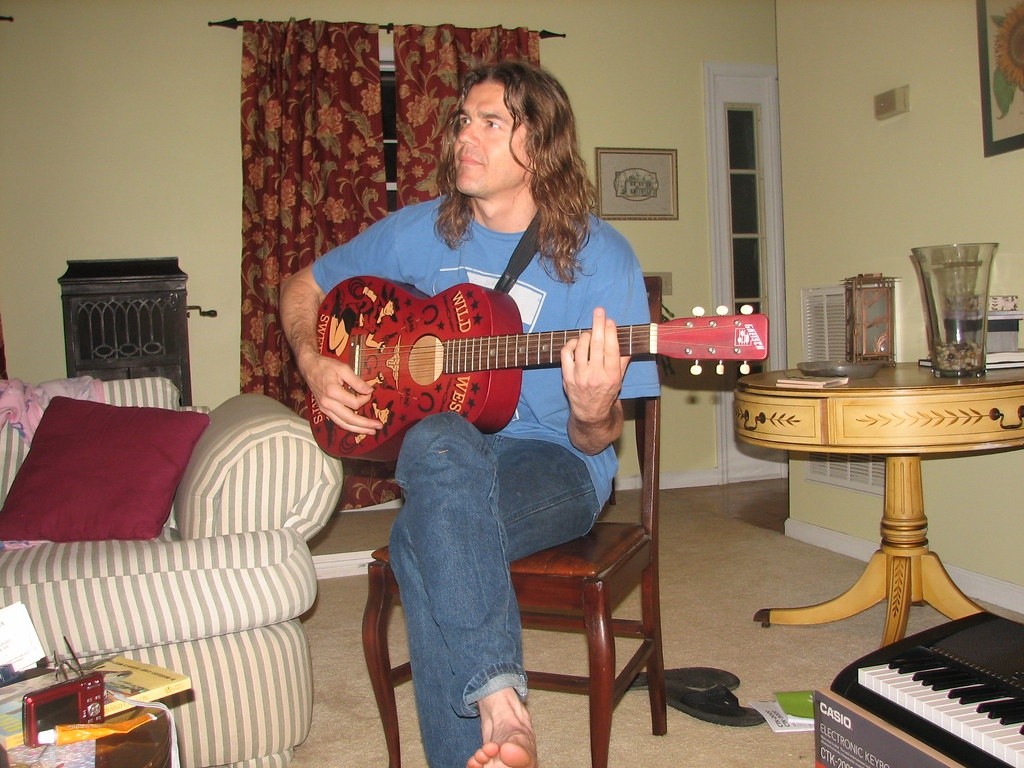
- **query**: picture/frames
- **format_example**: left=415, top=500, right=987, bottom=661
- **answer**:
left=975, top=0, right=1024, bottom=158
left=594, top=147, right=679, bottom=221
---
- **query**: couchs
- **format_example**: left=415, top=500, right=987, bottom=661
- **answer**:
left=0, top=378, right=342, bottom=768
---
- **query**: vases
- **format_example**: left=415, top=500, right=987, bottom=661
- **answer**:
left=909, top=241, right=999, bottom=381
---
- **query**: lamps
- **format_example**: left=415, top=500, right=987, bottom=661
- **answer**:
left=841, top=273, right=902, bottom=368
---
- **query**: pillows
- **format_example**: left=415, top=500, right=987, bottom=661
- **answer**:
left=1, top=396, right=211, bottom=542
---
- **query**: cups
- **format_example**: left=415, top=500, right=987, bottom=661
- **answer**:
left=911, top=242, right=1001, bottom=378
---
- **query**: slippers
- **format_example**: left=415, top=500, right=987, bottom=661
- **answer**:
left=626, top=666, right=740, bottom=693
left=667, top=684, right=766, bottom=726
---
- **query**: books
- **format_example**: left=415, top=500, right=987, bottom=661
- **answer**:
left=748, top=690, right=816, bottom=733
left=776, top=375, right=849, bottom=389
left=0, top=655, right=192, bottom=750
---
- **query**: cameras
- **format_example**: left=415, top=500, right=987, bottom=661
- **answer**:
left=22, top=672, right=105, bottom=748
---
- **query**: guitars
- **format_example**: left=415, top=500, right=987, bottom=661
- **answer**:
left=305, top=275, right=770, bottom=463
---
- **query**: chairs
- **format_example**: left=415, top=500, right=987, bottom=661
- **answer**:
left=359, top=278, right=673, bottom=767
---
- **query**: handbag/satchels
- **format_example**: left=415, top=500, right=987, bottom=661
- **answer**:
left=3, top=689, right=179, bottom=768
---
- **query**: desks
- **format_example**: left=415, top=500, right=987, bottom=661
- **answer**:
left=733, top=363, right=1024, bottom=655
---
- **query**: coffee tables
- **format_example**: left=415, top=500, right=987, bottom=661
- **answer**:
left=0, top=667, right=173, bottom=768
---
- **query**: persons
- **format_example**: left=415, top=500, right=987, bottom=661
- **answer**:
left=277, top=55, right=662, bottom=768
